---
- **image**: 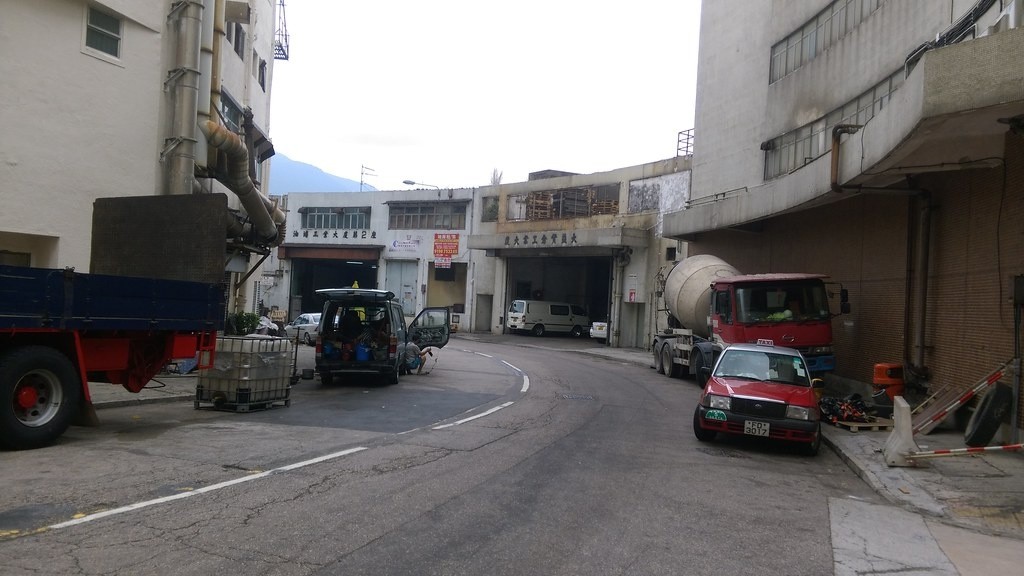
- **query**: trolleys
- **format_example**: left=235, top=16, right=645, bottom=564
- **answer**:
left=193, top=327, right=305, bottom=414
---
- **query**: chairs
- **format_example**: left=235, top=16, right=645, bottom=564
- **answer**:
left=771, top=357, right=798, bottom=385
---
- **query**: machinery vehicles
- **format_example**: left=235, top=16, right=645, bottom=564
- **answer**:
left=651, top=253, right=848, bottom=389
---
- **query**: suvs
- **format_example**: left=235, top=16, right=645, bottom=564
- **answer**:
left=506, top=299, right=591, bottom=341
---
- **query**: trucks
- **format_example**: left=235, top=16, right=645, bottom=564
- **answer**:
left=0, top=263, right=225, bottom=451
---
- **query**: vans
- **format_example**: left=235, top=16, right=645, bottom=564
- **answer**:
left=314, top=281, right=451, bottom=384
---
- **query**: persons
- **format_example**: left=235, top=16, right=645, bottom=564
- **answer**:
left=405, top=334, right=431, bottom=375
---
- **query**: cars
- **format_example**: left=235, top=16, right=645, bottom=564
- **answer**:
left=590, top=321, right=610, bottom=340
left=285, top=312, right=339, bottom=344
left=693, top=339, right=825, bottom=459
